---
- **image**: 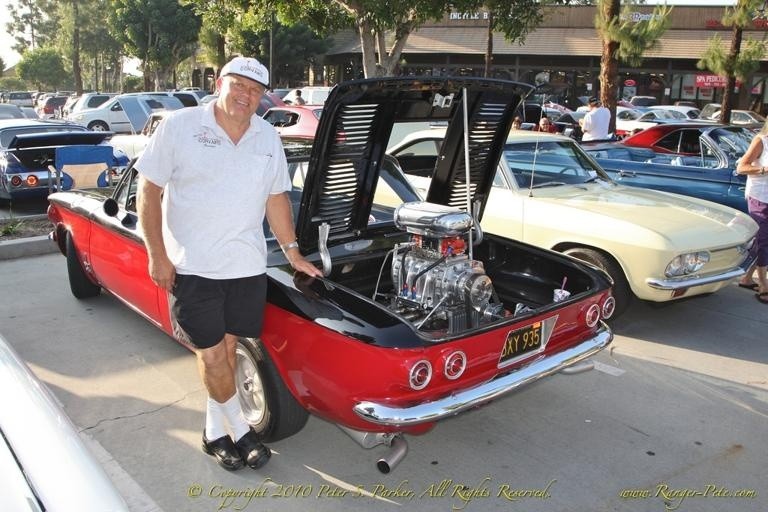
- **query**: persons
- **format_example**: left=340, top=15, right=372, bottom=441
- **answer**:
left=511, top=116, right=522, bottom=129
left=533, top=117, right=558, bottom=134
left=131, top=56, right=324, bottom=472
left=579, top=96, right=611, bottom=141
left=736, top=115, right=768, bottom=305
left=293, top=90, right=306, bottom=105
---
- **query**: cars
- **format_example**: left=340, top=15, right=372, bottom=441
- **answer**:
left=0, top=120, right=131, bottom=206
left=516, top=95, right=766, bottom=143
left=45, top=74, right=614, bottom=474
left=623, top=121, right=727, bottom=156
left=286, top=124, right=761, bottom=320
left=0, top=85, right=359, bottom=151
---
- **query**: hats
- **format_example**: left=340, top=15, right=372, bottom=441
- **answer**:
left=587, top=97, right=597, bottom=106
left=220, top=57, right=271, bottom=88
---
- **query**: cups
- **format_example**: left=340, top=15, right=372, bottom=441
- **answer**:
left=553, top=289, right=571, bottom=303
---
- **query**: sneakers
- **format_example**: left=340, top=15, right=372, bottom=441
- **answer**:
left=234, top=424, right=272, bottom=470
left=202, top=428, right=246, bottom=471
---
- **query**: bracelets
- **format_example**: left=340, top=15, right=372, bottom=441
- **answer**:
left=760, top=166, right=765, bottom=175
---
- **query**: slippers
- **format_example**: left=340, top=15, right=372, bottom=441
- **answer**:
left=738, top=283, right=759, bottom=292
left=755, top=292, right=767, bottom=304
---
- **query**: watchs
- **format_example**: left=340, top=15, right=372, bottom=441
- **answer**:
left=282, top=240, right=299, bottom=253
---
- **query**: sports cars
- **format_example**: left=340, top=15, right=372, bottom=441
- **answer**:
left=579, top=125, right=767, bottom=212
